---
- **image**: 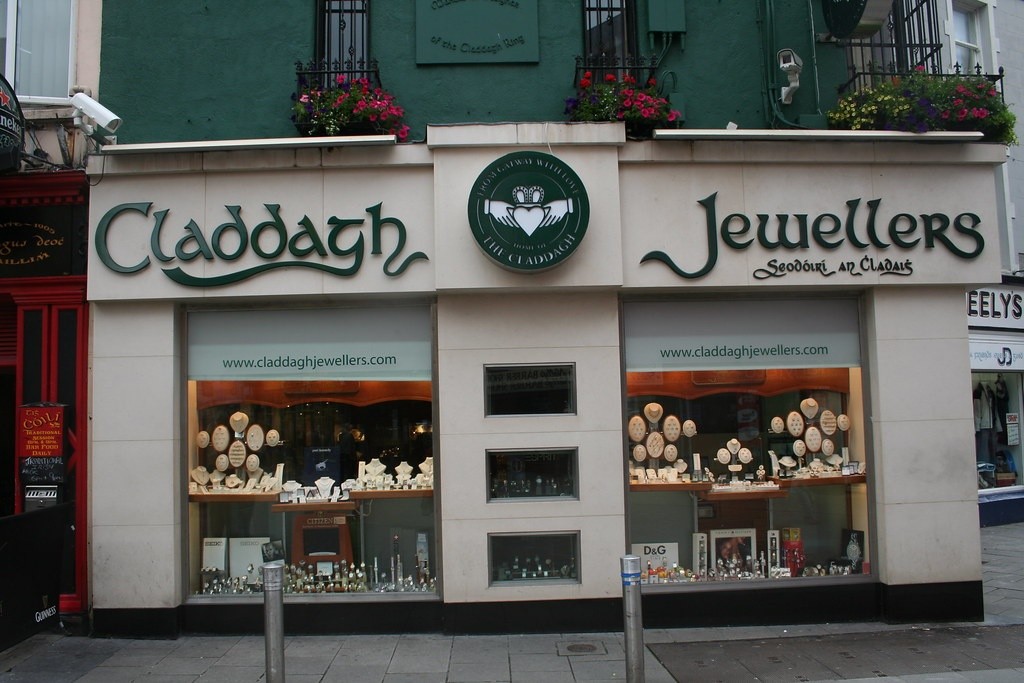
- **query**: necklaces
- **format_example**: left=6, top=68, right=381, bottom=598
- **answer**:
left=233, top=421, right=245, bottom=430
left=195, top=470, right=277, bottom=492
left=369, top=463, right=435, bottom=487
left=782, top=448, right=849, bottom=474
left=731, top=445, right=739, bottom=452
left=630, top=460, right=685, bottom=481
left=804, top=406, right=817, bottom=418
left=286, top=479, right=356, bottom=502
left=649, top=410, right=659, bottom=418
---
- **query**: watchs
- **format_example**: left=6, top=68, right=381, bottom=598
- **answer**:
left=847, top=532, right=861, bottom=570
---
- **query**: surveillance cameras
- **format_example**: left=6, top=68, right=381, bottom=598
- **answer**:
left=777, top=46, right=804, bottom=105
left=70, top=92, right=124, bottom=146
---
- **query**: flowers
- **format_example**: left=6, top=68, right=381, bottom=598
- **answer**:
left=288, top=75, right=411, bottom=146
left=562, top=68, right=683, bottom=140
left=820, top=60, right=1022, bottom=148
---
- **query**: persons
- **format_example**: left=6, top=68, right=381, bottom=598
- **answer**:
left=263, top=544, right=284, bottom=562
left=716, top=537, right=751, bottom=572
left=335, top=429, right=361, bottom=495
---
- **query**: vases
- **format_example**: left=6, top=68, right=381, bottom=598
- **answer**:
left=297, top=121, right=383, bottom=134
left=624, top=115, right=678, bottom=141
